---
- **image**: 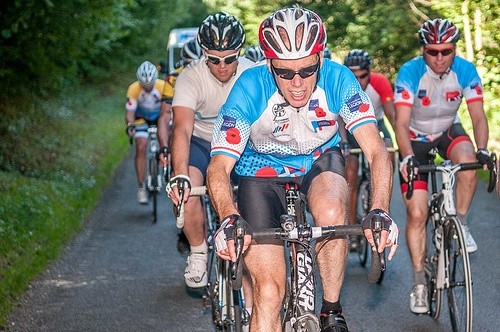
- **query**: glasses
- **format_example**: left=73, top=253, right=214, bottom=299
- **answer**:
left=271, top=58, right=320, bottom=79
left=356, top=74, right=368, bottom=78
left=204, top=51, right=240, bottom=64
left=424, top=48, right=455, bottom=56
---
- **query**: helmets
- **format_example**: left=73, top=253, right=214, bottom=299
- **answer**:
left=197, top=12, right=246, bottom=51
left=418, top=18, right=460, bottom=45
left=246, top=47, right=267, bottom=62
left=136, top=61, right=156, bottom=90
left=259, top=7, right=328, bottom=58
left=179, top=38, right=203, bottom=63
left=344, top=49, right=371, bottom=68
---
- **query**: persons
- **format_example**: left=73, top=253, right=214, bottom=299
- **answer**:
left=157, top=39, right=203, bottom=254
left=125, top=61, right=173, bottom=203
left=394, top=18, right=491, bottom=313
left=324, top=48, right=351, bottom=157
left=166, top=12, right=256, bottom=288
left=207, top=7, right=400, bottom=332
left=245, top=46, right=265, bottom=62
left=343, top=48, right=395, bottom=251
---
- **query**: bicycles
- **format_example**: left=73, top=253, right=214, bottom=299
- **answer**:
left=129, top=119, right=399, bottom=332
left=223, top=183, right=390, bottom=332
left=405, top=152, right=497, bottom=332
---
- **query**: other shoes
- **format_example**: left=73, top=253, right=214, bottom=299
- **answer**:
left=136, top=188, right=148, bottom=203
left=184, top=248, right=207, bottom=288
left=349, top=234, right=358, bottom=249
left=240, top=308, right=253, bottom=332
left=177, top=229, right=191, bottom=254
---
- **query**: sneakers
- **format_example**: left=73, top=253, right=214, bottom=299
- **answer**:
left=320, top=308, right=348, bottom=332
left=410, top=284, right=430, bottom=313
left=462, top=225, right=477, bottom=253
